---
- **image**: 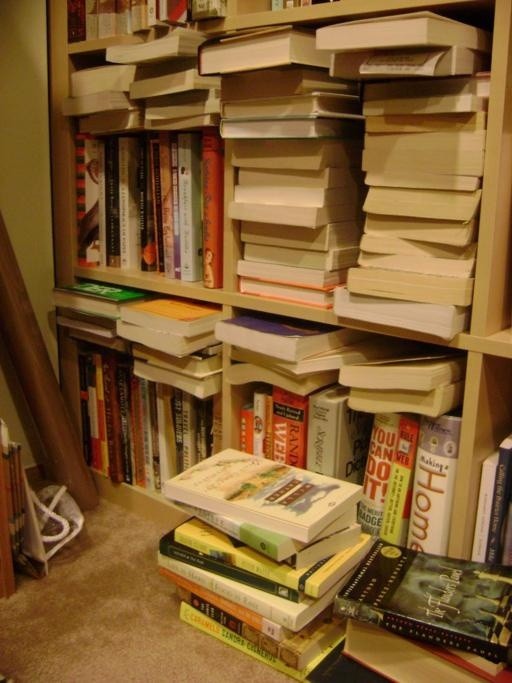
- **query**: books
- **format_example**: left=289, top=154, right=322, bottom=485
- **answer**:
left=337, top=539, right=511, bottom=683
left=83, top=0, right=220, bottom=39
left=473, top=435, right=512, bottom=565
left=2, top=420, right=25, bottom=600
left=50, top=283, right=224, bottom=491
left=272, top=1, right=343, bottom=13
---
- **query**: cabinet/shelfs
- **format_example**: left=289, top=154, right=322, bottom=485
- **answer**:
left=46, top=0, right=512, bottom=563
